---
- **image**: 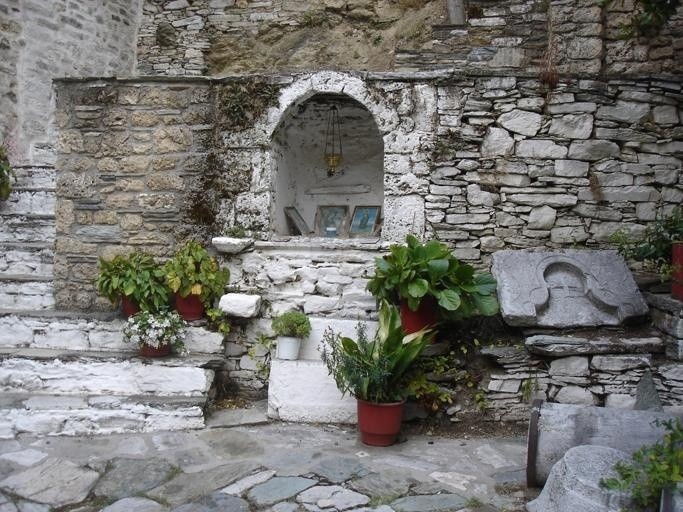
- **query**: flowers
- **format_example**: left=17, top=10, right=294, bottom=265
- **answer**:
left=121, top=309, right=191, bottom=357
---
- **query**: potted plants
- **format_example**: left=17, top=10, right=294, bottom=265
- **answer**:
left=363, top=235, right=498, bottom=336
left=161, top=239, right=231, bottom=321
left=607, top=205, right=683, bottom=302
left=598, top=418, right=683, bottom=512
left=313, top=299, right=442, bottom=445
left=97, top=246, right=162, bottom=318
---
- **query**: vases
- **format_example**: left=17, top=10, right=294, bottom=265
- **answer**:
left=137, top=339, right=172, bottom=358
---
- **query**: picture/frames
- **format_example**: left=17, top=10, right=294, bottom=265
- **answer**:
left=349, top=205, right=381, bottom=236
left=372, top=218, right=384, bottom=236
left=283, top=206, right=310, bottom=235
left=313, top=205, right=350, bottom=236
